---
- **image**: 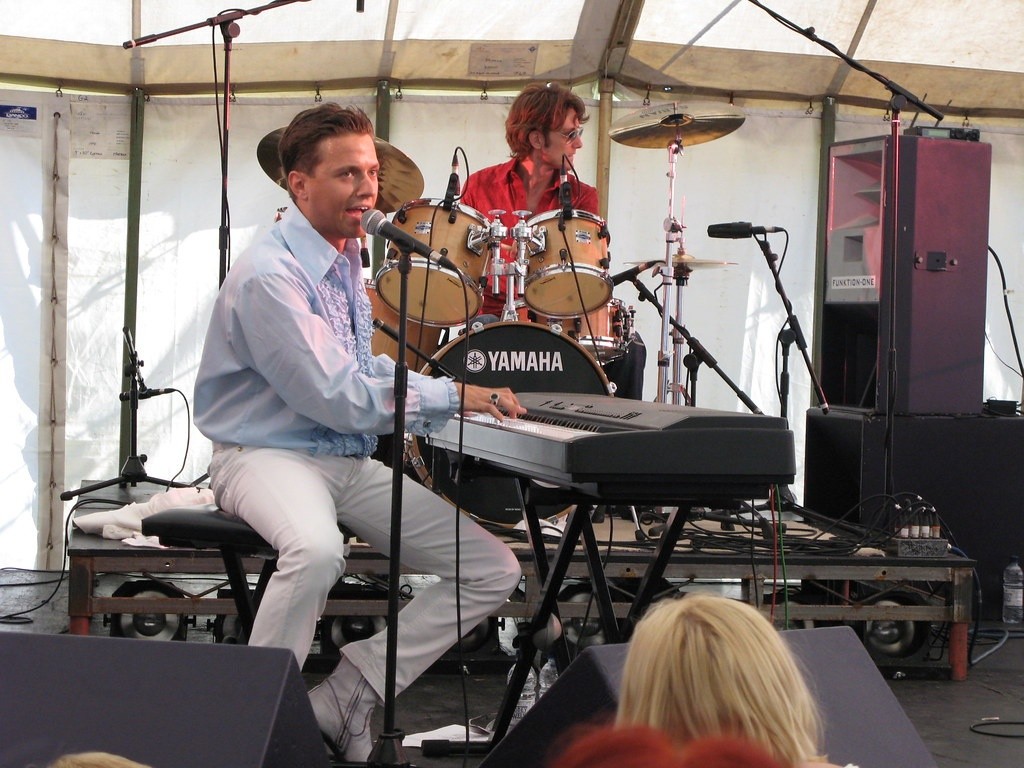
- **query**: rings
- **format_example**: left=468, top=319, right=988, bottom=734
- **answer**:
left=490, top=393, right=499, bottom=406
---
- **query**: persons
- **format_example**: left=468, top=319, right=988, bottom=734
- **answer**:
left=552, top=592, right=821, bottom=768
left=459, top=81, right=651, bottom=518
left=192, top=102, right=528, bottom=763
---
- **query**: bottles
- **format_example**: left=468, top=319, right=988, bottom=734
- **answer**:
left=505, top=656, right=537, bottom=736
left=538, top=658, right=559, bottom=701
left=1002, top=555, right=1024, bottom=629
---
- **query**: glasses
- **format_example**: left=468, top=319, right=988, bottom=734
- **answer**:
left=552, top=128, right=584, bottom=145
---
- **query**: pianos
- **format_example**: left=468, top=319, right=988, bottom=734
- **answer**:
left=422, top=392, right=799, bottom=762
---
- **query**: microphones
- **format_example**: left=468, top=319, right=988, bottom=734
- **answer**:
left=558, top=164, right=569, bottom=204
left=120, top=388, right=175, bottom=402
left=609, top=261, right=656, bottom=286
left=707, top=221, right=785, bottom=239
left=359, top=209, right=457, bottom=273
left=452, top=154, right=461, bottom=195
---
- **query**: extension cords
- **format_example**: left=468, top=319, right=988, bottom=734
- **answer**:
left=885, top=535, right=950, bottom=557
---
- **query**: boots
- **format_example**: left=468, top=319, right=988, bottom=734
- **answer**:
left=304, top=655, right=373, bottom=763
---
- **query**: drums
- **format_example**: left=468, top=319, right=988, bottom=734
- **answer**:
left=405, top=321, right=616, bottom=534
left=527, top=209, right=615, bottom=317
left=375, top=198, right=494, bottom=327
left=363, top=276, right=443, bottom=374
left=535, top=298, right=633, bottom=366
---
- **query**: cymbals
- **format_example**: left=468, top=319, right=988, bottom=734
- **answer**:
left=607, top=100, right=746, bottom=149
left=256, top=126, right=425, bottom=213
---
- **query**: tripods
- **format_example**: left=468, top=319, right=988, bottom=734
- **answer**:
left=60, top=325, right=193, bottom=502
left=688, top=236, right=873, bottom=539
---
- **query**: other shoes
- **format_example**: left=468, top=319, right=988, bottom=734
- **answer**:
left=514, top=514, right=581, bottom=544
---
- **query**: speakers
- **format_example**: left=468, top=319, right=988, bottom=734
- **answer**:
left=0, top=630, right=333, bottom=768
left=475, top=626, right=938, bottom=768
left=805, top=407, right=1024, bottom=619
left=811, top=133, right=992, bottom=414
